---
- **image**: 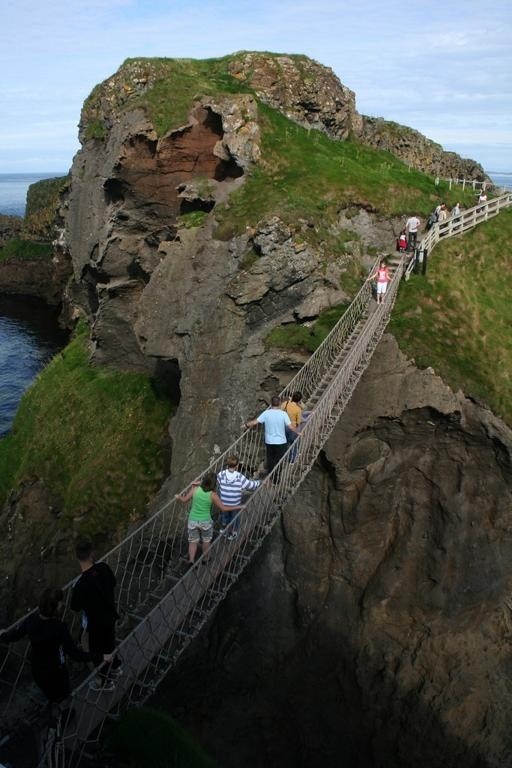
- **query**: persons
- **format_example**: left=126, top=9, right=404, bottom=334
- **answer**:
left=239, top=396, right=305, bottom=486
left=403, top=213, right=421, bottom=252
left=0, top=586, right=114, bottom=724
left=398, top=232, right=407, bottom=253
left=174, top=472, right=247, bottom=569
left=69, top=535, right=123, bottom=692
left=298, top=399, right=312, bottom=417
left=281, top=390, right=302, bottom=465
left=425, top=202, right=460, bottom=237
left=475, top=188, right=483, bottom=196
left=191, top=456, right=266, bottom=540
left=478, top=191, right=488, bottom=211
left=367, top=261, right=393, bottom=305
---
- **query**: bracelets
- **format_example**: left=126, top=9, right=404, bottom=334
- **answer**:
left=245, top=424, right=249, bottom=428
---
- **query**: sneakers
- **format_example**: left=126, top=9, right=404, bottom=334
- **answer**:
left=269, top=472, right=279, bottom=486
left=89, top=667, right=123, bottom=691
left=188, top=556, right=211, bottom=569
left=220, top=528, right=237, bottom=539
left=377, top=301, right=385, bottom=305
